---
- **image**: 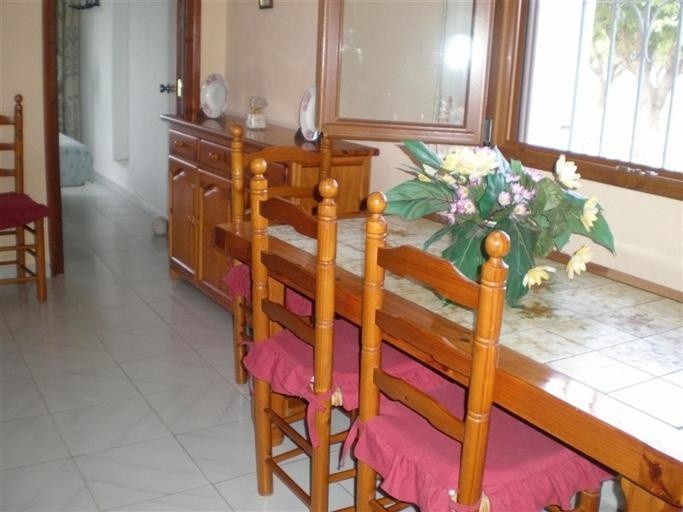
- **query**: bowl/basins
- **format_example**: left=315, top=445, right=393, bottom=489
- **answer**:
left=199, top=72, right=228, bottom=120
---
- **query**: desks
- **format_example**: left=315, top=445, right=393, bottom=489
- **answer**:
left=210, top=196, right=681, bottom=512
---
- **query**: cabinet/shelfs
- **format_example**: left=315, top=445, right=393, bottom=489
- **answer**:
left=161, top=109, right=379, bottom=317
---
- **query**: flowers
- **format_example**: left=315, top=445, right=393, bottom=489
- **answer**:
left=384, top=132, right=618, bottom=314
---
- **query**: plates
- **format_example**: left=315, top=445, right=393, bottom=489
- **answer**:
left=298, top=88, right=322, bottom=143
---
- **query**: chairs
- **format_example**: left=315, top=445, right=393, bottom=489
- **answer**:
left=1, top=92, right=56, bottom=302
left=355, top=190, right=616, bottom=512
left=244, top=155, right=451, bottom=511
left=226, top=126, right=346, bottom=387
